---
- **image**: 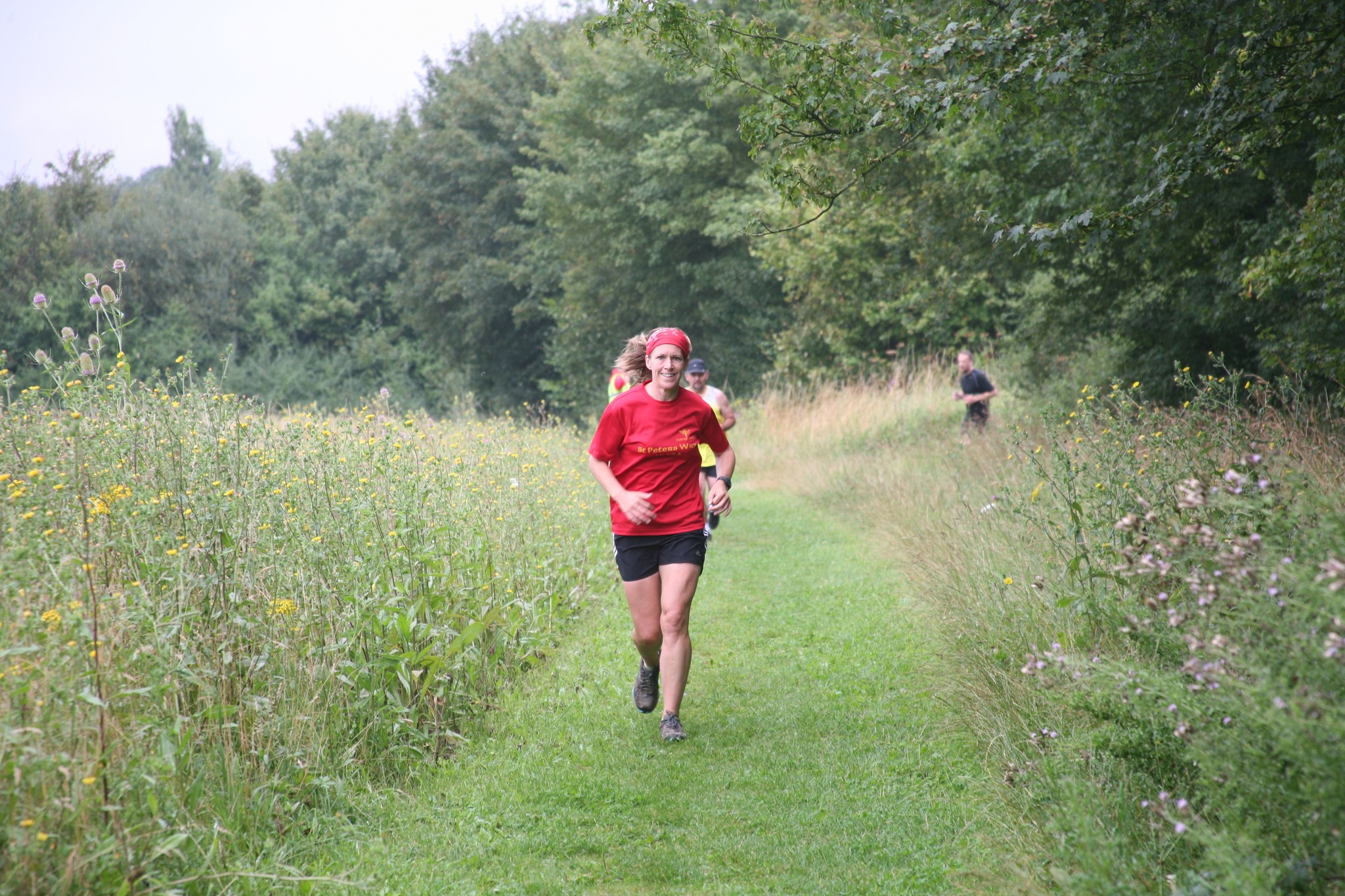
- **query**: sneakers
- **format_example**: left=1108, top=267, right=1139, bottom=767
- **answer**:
left=631, top=650, right=661, bottom=714
left=658, top=710, right=685, bottom=741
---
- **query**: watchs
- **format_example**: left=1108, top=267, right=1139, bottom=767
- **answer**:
left=715, top=476, right=733, bottom=490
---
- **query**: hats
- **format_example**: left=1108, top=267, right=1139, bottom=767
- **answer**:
left=687, top=360, right=706, bottom=374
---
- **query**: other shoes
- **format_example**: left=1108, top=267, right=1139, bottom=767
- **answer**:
left=708, top=512, right=719, bottom=528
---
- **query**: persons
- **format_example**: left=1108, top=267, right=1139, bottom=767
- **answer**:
left=951, top=351, right=997, bottom=438
left=587, top=326, right=736, bottom=744
left=680, top=361, right=736, bottom=542
left=607, top=332, right=652, bottom=403
